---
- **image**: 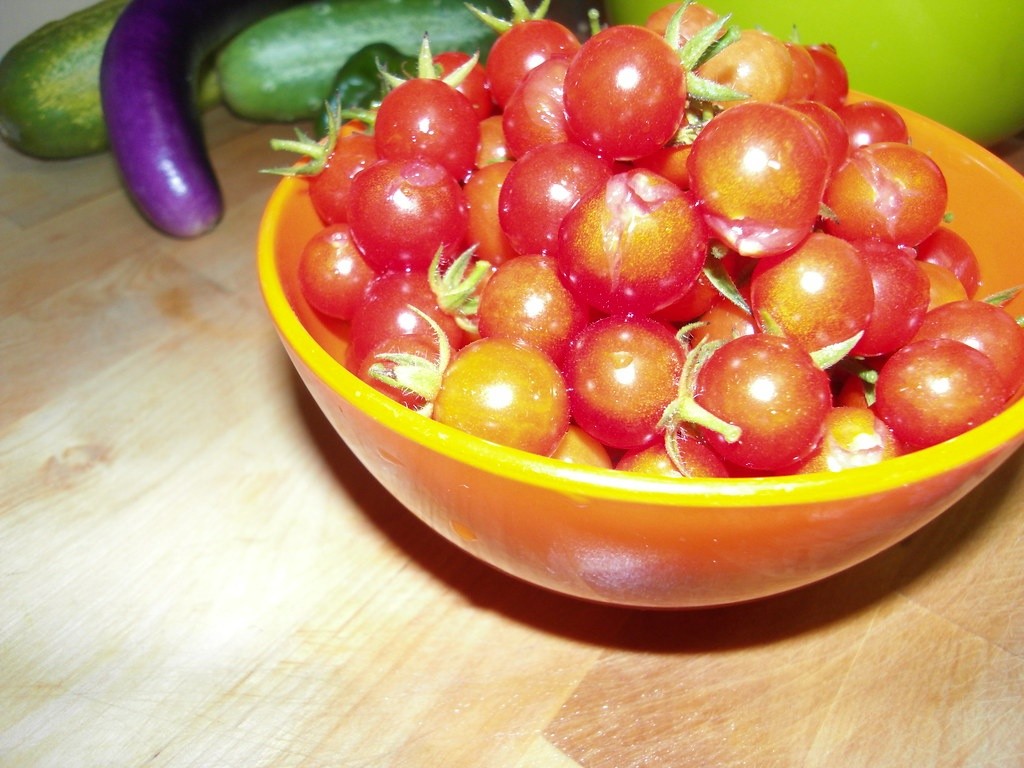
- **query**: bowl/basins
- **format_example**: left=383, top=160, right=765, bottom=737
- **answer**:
left=253, top=86, right=1024, bottom=609
left=602, top=0, right=1024, bottom=150
